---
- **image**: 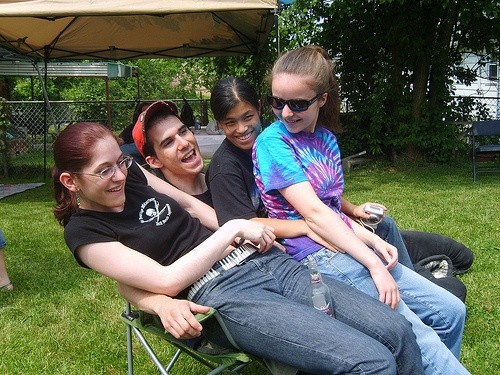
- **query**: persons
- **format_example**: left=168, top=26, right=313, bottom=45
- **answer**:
left=205, top=76, right=414, bottom=273
left=251, top=45, right=473, bottom=375
left=116, top=100, right=473, bottom=341
left=0, top=228, right=13, bottom=292
left=51, top=121, right=424, bottom=375
left=116, top=102, right=151, bottom=145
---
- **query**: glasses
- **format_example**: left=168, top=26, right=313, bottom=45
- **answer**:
left=268, top=92, right=326, bottom=111
left=71, top=156, right=132, bottom=181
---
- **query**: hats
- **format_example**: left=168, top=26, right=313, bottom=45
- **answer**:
left=132, top=100, right=178, bottom=159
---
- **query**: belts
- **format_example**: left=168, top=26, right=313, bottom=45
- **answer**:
left=186, top=243, right=259, bottom=301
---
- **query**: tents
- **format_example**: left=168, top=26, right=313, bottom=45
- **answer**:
left=0, top=0, right=296, bottom=182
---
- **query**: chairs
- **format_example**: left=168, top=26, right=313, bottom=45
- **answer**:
left=120, top=300, right=297, bottom=375
left=472, top=120, right=500, bottom=183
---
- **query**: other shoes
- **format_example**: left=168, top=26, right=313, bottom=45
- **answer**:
left=0, top=282, right=14, bottom=291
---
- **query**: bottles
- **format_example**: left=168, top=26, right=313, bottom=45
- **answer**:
left=307, top=262, right=334, bottom=319
left=194, top=115, right=201, bottom=134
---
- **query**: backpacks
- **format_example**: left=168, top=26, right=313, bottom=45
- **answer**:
left=181, top=98, right=195, bottom=126
